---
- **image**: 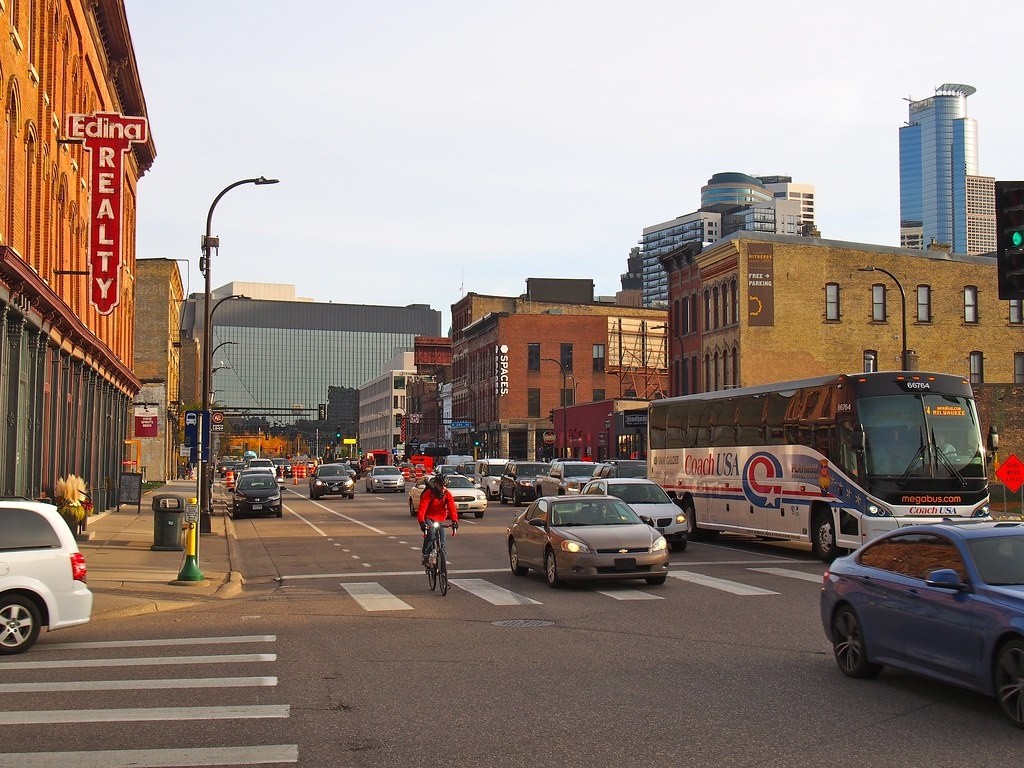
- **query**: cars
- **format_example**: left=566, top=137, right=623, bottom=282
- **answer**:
left=365, top=466, right=405, bottom=493
left=430, top=454, right=477, bottom=485
left=817, top=519, right=1023, bottom=730
left=216, top=457, right=292, bottom=519
left=408, top=473, right=487, bottom=518
left=506, top=494, right=670, bottom=588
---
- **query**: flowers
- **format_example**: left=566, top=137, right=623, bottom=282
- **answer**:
left=54, top=473, right=94, bottom=524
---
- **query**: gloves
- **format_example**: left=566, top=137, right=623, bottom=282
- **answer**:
left=420, top=524, right=428, bottom=532
left=451, top=521, right=458, bottom=530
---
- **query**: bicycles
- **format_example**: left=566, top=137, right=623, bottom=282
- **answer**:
left=421, top=521, right=457, bottom=597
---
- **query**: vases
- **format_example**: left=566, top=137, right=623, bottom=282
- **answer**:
left=64, top=515, right=77, bottom=540
left=80, top=512, right=87, bottom=531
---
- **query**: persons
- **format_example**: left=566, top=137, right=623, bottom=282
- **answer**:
left=936, top=432, right=957, bottom=457
left=417, top=473, right=458, bottom=559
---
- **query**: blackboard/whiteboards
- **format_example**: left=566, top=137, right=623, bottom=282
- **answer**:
left=117, top=473, right=141, bottom=504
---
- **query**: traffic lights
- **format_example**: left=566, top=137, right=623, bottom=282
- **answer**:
left=992, top=179, right=1023, bottom=301
left=336, top=426, right=341, bottom=444
left=473, top=434, right=480, bottom=447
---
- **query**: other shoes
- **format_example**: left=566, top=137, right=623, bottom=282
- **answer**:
left=422, top=560, right=431, bottom=566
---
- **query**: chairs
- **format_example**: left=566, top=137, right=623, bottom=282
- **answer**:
left=263, top=479, right=271, bottom=487
left=574, top=506, right=597, bottom=522
left=243, top=481, right=251, bottom=488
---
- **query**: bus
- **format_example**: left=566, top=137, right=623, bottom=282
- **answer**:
left=646, top=371, right=1000, bottom=565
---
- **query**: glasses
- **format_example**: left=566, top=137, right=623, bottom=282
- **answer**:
left=437, top=483, right=448, bottom=487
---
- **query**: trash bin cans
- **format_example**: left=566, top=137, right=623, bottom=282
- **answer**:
left=151, top=494, right=186, bottom=552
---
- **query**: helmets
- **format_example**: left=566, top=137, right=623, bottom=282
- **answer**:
left=435, top=474, right=449, bottom=484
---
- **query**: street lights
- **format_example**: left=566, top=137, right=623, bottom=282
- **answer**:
left=373, top=412, right=387, bottom=449
left=196, top=176, right=281, bottom=533
left=568, top=374, right=581, bottom=404
left=651, top=325, right=686, bottom=396
left=209, top=294, right=252, bottom=514
left=856, top=265, right=907, bottom=371
left=539, top=357, right=567, bottom=459
left=463, top=386, right=480, bottom=462
left=392, top=406, right=407, bottom=463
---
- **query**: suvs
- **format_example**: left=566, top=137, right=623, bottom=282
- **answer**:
left=473, top=457, right=517, bottom=501
left=589, top=458, right=646, bottom=491
left=333, top=457, right=361, bottom=483
left=0, top=494, right=94, bottom=656
left=499, top=458, right=549, bottom=506
left=576, top=477, right=690, bottom=553
left=533, top=457, right=600, bottom=498
left=308, top=464, right=355, bottom=500
